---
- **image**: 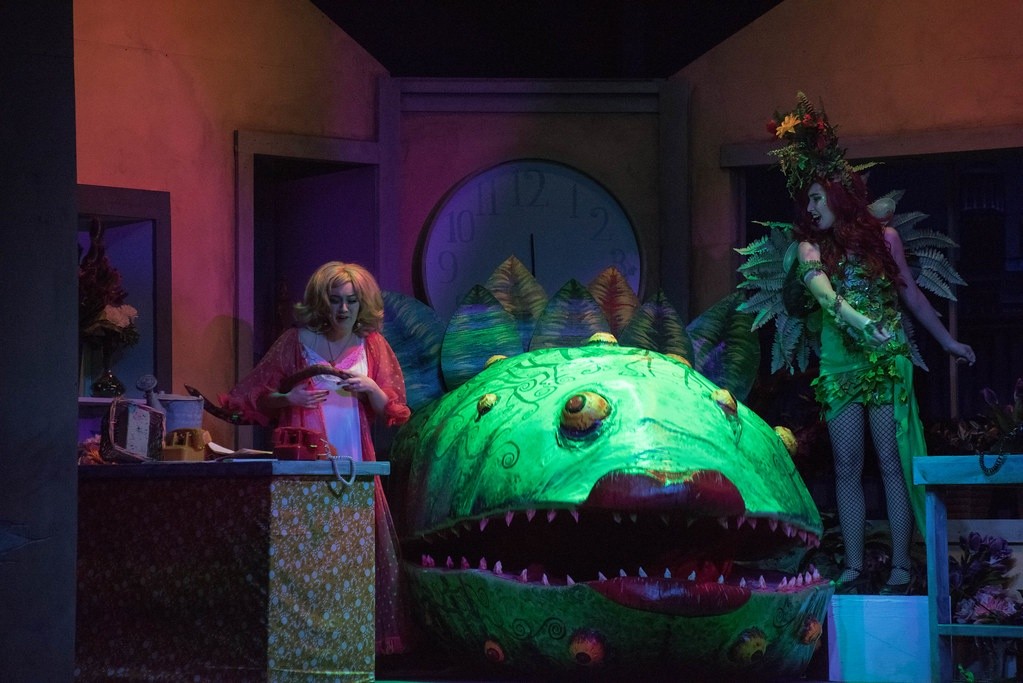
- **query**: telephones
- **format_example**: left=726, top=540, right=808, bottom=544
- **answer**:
left=273, top=426, right=338, bottom=461
left=163, top=428, right=212, bottom=462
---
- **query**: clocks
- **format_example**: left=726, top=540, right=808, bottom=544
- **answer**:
left=412, top=156, right=645, bottom=320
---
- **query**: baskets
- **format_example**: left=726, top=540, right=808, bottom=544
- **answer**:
left=101, top=397, right=166, bottom=462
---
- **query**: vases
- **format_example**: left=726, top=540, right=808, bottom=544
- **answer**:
left=93, top=340, right=128, bottom=399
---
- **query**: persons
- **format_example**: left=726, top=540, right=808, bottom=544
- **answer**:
left=796, top=172, right=976, bottom=594
left=229, top=262, right=410, bottom=656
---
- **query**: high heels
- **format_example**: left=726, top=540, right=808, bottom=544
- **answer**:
left=879, top=566, right=916, bottom=595
left=834, top=567, right=866, bottom=595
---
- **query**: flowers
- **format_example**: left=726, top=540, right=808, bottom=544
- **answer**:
left=908, top=533, right=1023, bottom=683
left=74, top=249, right=142, bottom=345
